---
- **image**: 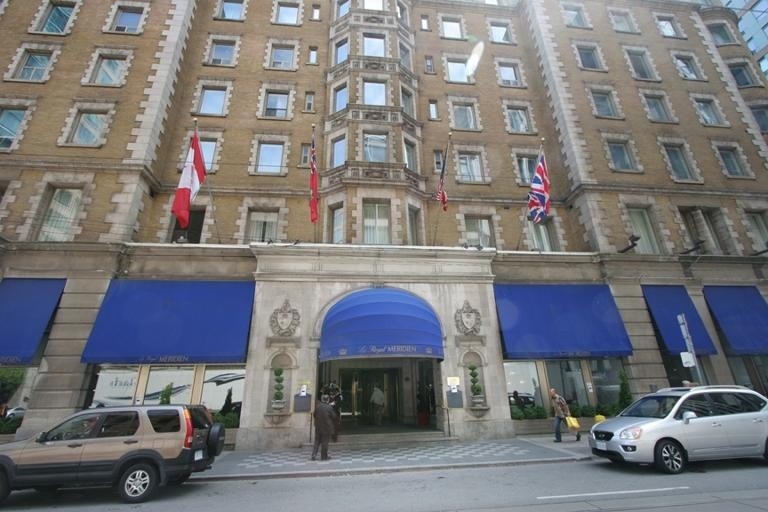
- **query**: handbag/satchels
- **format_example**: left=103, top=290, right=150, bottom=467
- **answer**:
left=565, top=416, right=580, bottom=430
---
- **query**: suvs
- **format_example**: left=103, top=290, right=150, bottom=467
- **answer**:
left=0, top=404, right=225, bottom=504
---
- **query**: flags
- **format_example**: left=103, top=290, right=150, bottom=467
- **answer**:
left=526, top=151, right=550, bottom=227
left=432, top=151, right=448, bottom=212
left=170, top=132, right=206, bottom=230
left=309, top=132, right=318, bottom=225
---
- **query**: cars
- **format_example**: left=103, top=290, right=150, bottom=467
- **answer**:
left=6, top=407, right=23, bottom=418
left=588, top=384, right=768, bottom=474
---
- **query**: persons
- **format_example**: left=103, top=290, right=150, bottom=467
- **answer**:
left=311, top=393, right=339, bottom=461
left=417, top=386, right=429, bottom=425
left=672, top=379, right=692, bottom=419
left=86, top=402, right=106, bottom=434
left=328, top=391, right=342, bottom=443
left=549, top=388, right=581, bottom=443
left=370, top=386, right=385, bottom=427
left=513, top=391, right=524, bottom=406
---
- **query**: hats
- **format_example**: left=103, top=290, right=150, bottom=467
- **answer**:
left=321, top=394, right=330, bottom=403
